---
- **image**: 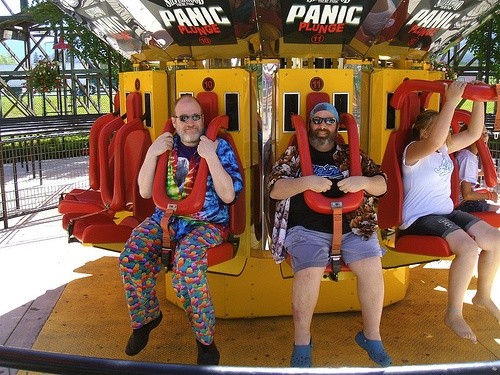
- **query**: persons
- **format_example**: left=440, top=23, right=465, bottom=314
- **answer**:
left=120, top=95, right=243, bottom=367
left=456, top=123, right=500, bottom=212
left=267, top=102, right=392, bottom=368
left=398, top=80, right=500, bottom=340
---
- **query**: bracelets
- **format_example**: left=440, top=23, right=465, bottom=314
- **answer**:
left=486, top=193, right=492, bottom=200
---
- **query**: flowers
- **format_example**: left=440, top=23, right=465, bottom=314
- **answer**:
left=22, top=55, right=67, bottom=94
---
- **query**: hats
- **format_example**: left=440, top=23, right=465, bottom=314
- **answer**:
left=310, top=102, right=339, bottom=123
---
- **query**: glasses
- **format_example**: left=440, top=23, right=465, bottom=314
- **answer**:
left=174, top=114, right=202, bottom=123
left=311, top=117, right=335, bottom=125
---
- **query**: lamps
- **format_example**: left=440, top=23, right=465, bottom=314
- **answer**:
left=52, top=37, right=70, bottom=49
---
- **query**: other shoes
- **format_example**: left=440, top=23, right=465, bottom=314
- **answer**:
left=125, top=310, right=163, bottom=356
left=196, top=339, right=220, bottom=365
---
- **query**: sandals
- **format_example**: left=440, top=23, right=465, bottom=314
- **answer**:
left=355, top=331, right=392, bottom=367
left=290, top=337, right=313, bottom=367
